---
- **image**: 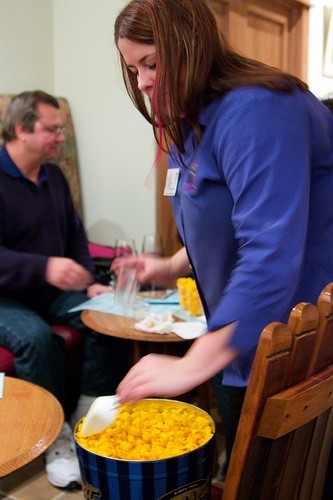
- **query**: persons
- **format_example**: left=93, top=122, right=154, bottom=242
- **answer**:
left=0, top=90, right=118, bottom=491
left=115, top=2, right=333, bottom=482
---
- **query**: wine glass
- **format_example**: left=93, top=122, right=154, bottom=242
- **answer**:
left=141, top=234, right=166, bottom=298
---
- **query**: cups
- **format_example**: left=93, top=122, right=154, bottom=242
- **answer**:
left=113, top=267, right=140, bottom=308
left=113, top=239, right=139, bottom=275
left=177, top=277, right=193, bottom=310
left=186, top=280, right=204, bottom=316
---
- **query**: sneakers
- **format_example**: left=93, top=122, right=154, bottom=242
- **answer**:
left=43, top=420, right=84, bottom=490
left=72, top=395, right=95, bottom=420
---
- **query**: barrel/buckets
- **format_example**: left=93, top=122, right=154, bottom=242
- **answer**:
left=73, top=398, right=216, bottom=500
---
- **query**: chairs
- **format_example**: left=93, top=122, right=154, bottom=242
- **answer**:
left=212, top=281, right=333, bottom=500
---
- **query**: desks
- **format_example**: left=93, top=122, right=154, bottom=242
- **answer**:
left=81, top=289, right=218, bottom=419
left=0, top=375, right=63, bottom=481
